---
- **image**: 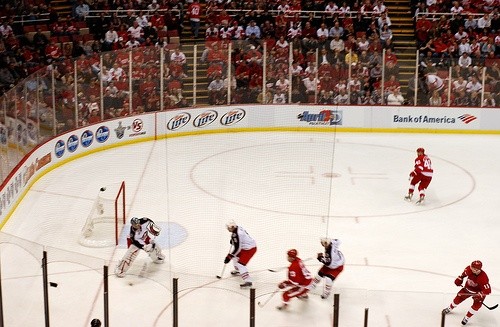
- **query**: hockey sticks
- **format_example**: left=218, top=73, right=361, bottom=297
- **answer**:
left=216, top=245, right=233, bottom=279
left=268, top=256, right=318, bottom=272
left=257, top=287, right=280, bottom=307
left=129, top=253, right=151, bottom=285
left=460, top=283, right=498, bottom=310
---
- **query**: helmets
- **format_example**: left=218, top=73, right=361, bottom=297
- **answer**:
left=131, top=217, right=140, bottom=225
left=417, top=148, right=424, bottom=152
left=91, top=319, right=101, bottom=327
left=288, top=249, right=296, bottom=257
left=321, top=237, right=331, bottom=247
left=225, top=220, right=237, bottom=228
left=472, top=260, right=482, bottom=271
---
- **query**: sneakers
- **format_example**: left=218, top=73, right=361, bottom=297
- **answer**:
left=240, top=282, right=252, bottom=288
left=320, top=291, right=330, bottom=299
left=461, top=317, right=468, bottom=325
left=231, top=270, right=240, bottom=276
left=297, top=295, right=307, bottom=300
left=278, top=304, right=287, bottom=311
left=443, top=306, right=452, bottom=314
left=416, top=198, right=423, bottom=205
left=405, top=193, right=413, bottom=200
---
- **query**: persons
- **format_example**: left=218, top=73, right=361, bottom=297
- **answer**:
left=200, top=0, right=405, bottom=105
left=274, top=249, right=313, bottom=310
left=310, top=236, right=344, bottom=298
left=184, top=0, right=202, bottom=39
left=115, top=217, right=166, bottom=278
left=442, top=260, right=491, bottom=326
left=0, top=0, right=188, bottom=152
left=414, top=0, right=500, bottom=107
left=225, top=218, right=257, bottom=289
left=403, top=147, right=434, bottom=205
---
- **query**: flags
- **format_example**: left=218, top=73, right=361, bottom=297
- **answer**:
left=25, top=118, right=38, bottom=147
left=16, top=118, right=27, bottom=154
left=0, top=123, right=8, bottom=159
left=5, top=115, right=17, bottom=152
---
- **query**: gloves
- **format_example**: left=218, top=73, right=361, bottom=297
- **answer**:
left=224, top=254, right=234, bottom=264
left=279, top=281, right=289, bottom=289
left=454, top=276, right=463, bottom=287
left=317, top=253, right=324, bottom=262
left=230, top=240, right=233, bottom=244
left=410, top=170, right=417, bottom=177
left=472, top=292, right=483, bottom=301
